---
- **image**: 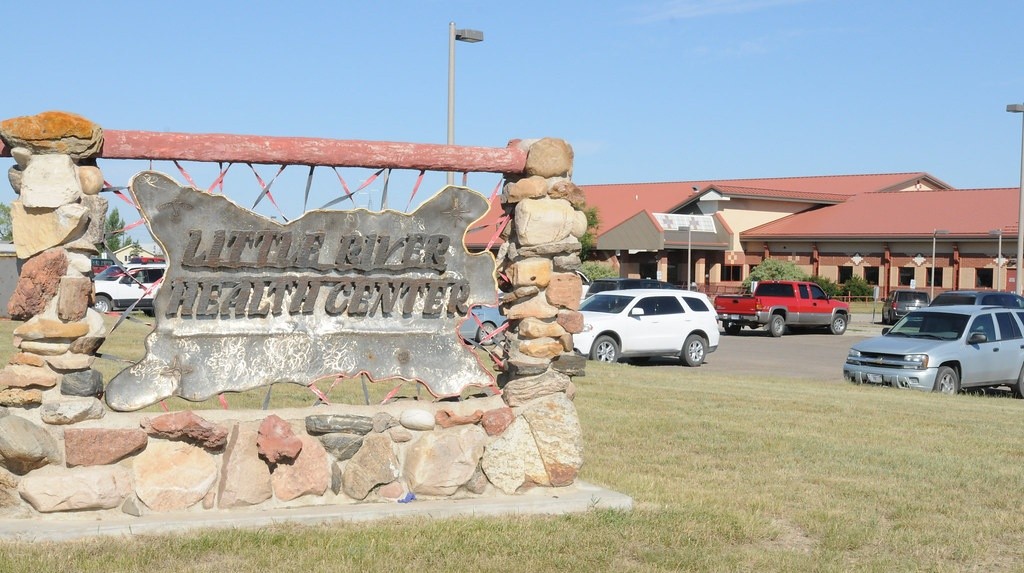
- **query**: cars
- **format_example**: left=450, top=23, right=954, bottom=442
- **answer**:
left=460, top=293, right=508, bottom=345
left=574, top=270, right=591, bottom=299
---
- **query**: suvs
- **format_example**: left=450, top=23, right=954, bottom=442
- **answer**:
left=571, top=289, right=720, bottom=369
left=843, top=304, right=1024, bottom=399
left=918, top=290, right=1024, bottom=342
left=880, top=289, right=931, bottom=325
left=584, top=277, right=679, bottom=312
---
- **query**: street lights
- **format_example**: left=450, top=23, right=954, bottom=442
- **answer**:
left=677, top=225, right=692, bottom=290
left=120, top=231, right=125, bottom=246
left=990, top=229, right=1002, bottom=292
left=1005, top=102, right=1024, bottom=306
left=930, top=227, right=949, bottom=303
left=446, top=21, right=484, bottom=185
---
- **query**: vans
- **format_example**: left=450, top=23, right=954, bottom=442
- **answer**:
left=128, top=257, right=165, bottom=265
left=92, top=262, right=166, bottom=317
left=90, top=258, right=115, bottom=274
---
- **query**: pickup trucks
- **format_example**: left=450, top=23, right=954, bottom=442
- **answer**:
left=714, top=280, right=851, bottom=337
